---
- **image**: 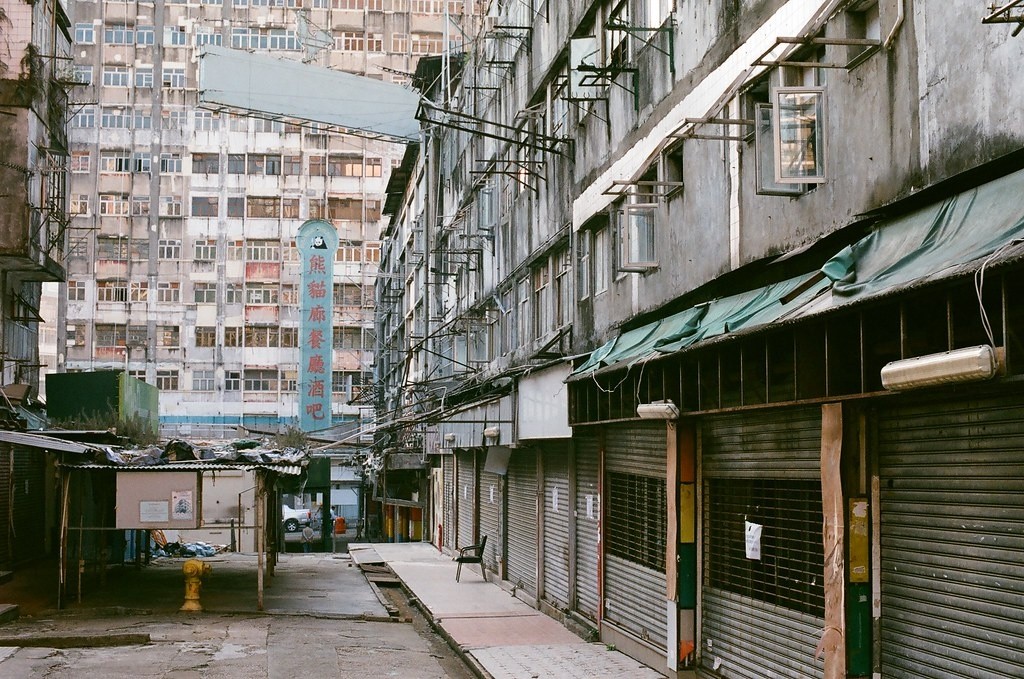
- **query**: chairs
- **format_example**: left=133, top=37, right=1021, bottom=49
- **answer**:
left=453, top=534, right=490, bottom=583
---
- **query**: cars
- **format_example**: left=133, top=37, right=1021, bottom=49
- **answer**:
left=281, top=504, right=311, bottom=533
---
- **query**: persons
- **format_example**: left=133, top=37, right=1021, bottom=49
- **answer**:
left=300, top=522, right=314, bottom=553
left=330, top=506, right=337, bottom=533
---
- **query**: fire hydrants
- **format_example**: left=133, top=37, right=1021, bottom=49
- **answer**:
left=178, top=558, right=215, bottom=618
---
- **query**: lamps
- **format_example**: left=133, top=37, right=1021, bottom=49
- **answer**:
left=483, top=427, right=500, bottom=438
left=444, top=433, right=455, bottom=441
left=879, top=343, right=1002, bottom=393
left=636, top=396, right=682, bottom=423
